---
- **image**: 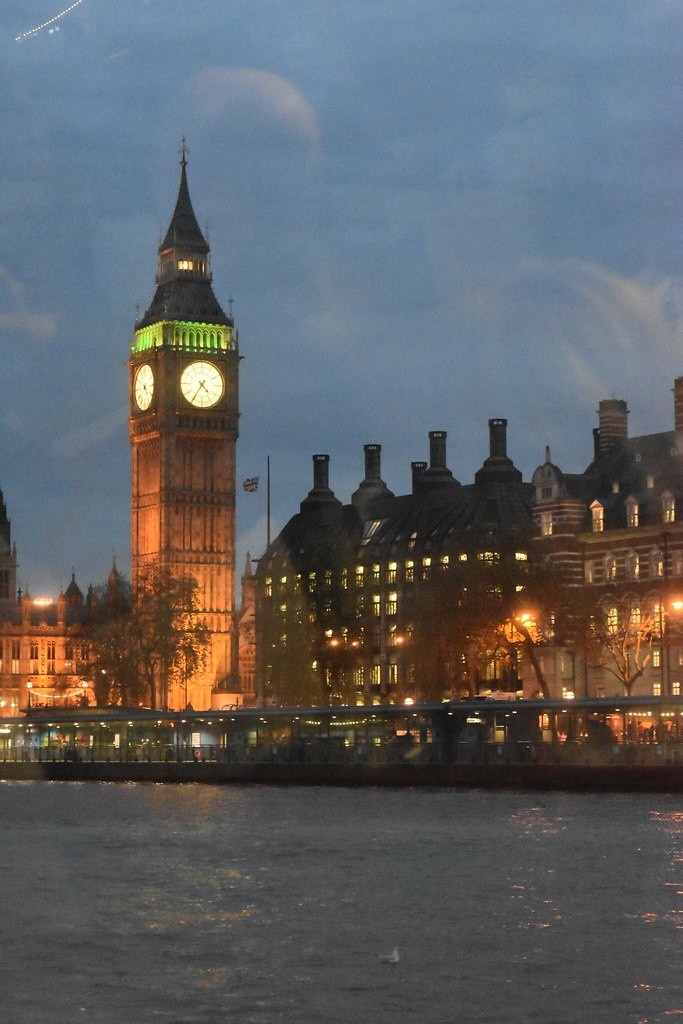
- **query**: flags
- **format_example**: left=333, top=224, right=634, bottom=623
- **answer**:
left=243, top=475, right=260, bottom=493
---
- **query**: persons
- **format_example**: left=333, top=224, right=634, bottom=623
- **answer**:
left=641, top=724, right=655, bottom=743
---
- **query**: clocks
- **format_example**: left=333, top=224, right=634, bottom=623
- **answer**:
left=179, top=360, right=226, bottom=410
left=133, top=364, right=155, bottom=411
